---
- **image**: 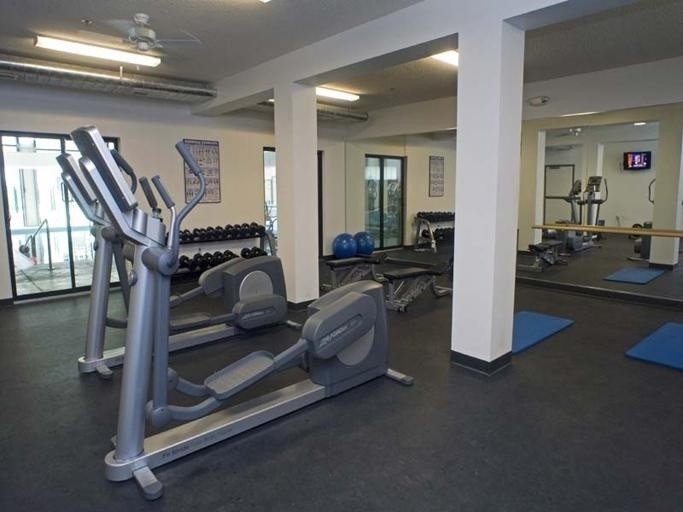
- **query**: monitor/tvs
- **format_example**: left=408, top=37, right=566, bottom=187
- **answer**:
left=624, top=151, right=652, bottom=170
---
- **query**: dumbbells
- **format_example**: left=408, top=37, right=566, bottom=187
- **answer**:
left=180, top=250, right=233, bottom=270
left=417, top=210, right=455, bottom=243
left=241, top=247, right=260, bottom=258
left=178, top=222, right=264, bottom=241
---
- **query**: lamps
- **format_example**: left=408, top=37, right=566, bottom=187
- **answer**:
left=312, top=87, right=361, bottom=102
left=28, top=36, right=162, bottom=69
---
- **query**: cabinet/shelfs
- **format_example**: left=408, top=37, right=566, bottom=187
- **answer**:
left=413, top=210, right=453, bottom=254
left=160, top=222, right=276, bottom=275
